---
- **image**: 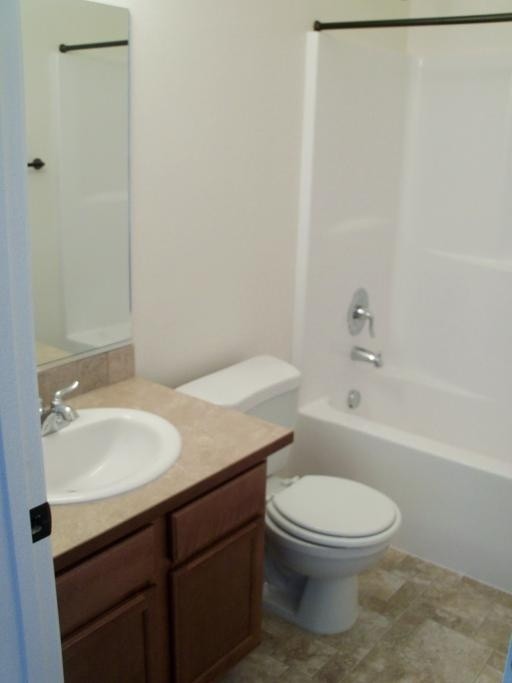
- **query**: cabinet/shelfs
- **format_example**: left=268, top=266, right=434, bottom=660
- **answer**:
left=170, top=464, right=266, bottom=679
left=61, top=513, right=171, bottom=680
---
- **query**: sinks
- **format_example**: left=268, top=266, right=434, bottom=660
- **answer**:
left=41, top=406, right=183, bottom=506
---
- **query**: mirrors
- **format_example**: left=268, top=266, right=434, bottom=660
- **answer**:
left=18, top=0, right=136, bottom=370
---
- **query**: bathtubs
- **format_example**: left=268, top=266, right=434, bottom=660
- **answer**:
left=297, top=366, right=512, bottom=597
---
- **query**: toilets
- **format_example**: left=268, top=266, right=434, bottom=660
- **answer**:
left=174, top=353, right=403, bottom=635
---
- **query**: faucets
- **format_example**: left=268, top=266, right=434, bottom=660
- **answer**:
left=354, top=346, right=382, bottom=368
left=39, top=378, right=80, bottom=429
